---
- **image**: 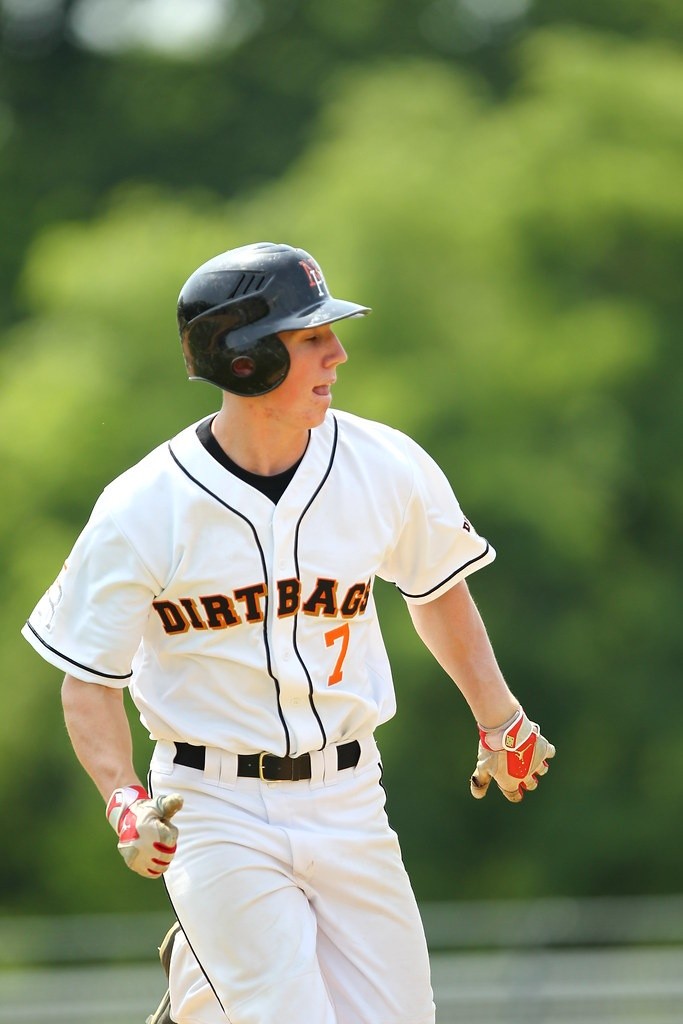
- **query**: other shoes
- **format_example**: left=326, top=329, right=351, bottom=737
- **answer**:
left=148, top=921, right=180, bottom=1024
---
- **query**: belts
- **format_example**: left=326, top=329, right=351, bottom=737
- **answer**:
left=174, top=739, right=361, bottom=783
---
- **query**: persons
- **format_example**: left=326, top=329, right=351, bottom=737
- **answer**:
left=22, top=241, right=555, bottom=1024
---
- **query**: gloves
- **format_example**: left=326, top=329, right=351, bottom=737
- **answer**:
left=104, top=786, right=184, bottom=878
left=471, top=705, right=557, bottom=804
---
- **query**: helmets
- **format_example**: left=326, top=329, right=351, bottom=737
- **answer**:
left=178, top=244, right=372, bottom=396
